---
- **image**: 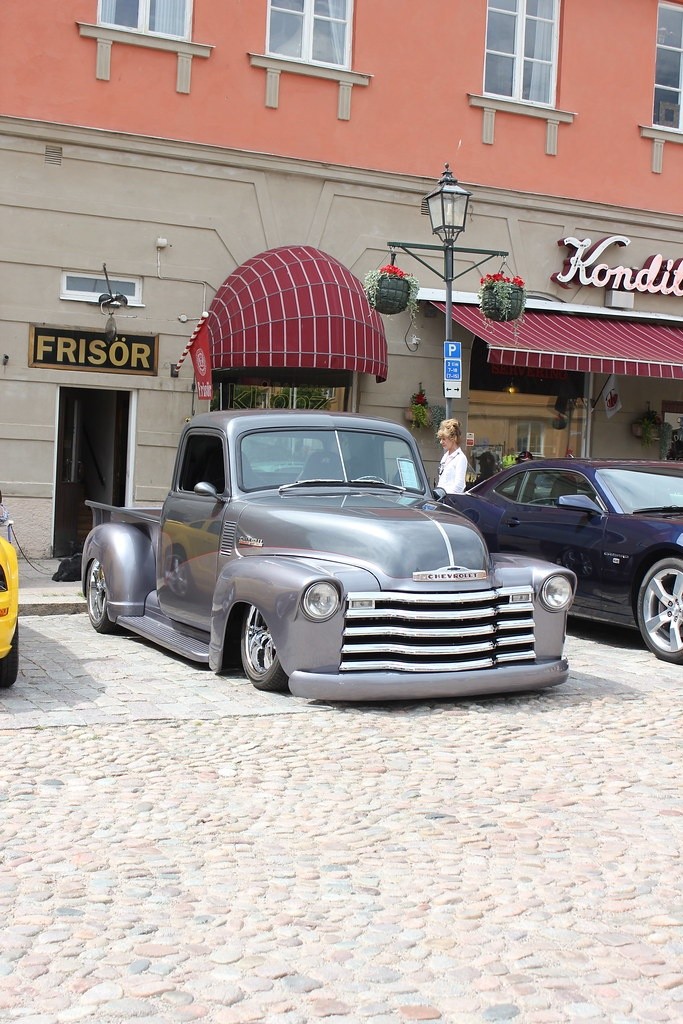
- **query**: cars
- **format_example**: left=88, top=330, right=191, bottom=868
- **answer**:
left=438, top=458, right=683, bottom=664
left=81, top=408, right=577, bottom=699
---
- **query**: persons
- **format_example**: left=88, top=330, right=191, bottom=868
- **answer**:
left=515, top=450, right=533, bottom=464
left=476, top=447, right=501, bottom=479
left=436, top=418, right=467, bottom=493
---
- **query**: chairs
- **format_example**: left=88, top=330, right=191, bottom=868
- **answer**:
left=202, top=447, right=254, bottom=493
left=551, top=476, right=577, bottom=500
left=299, top=450, right=345, bottom=482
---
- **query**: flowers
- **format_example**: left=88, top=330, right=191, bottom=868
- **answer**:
left=410, top=392, right=433, bottom=429
left=362, top=265, right=421, bottom=329
left=640, top=410, right=674, bottom=460
left=479, top=272, right=528, bottom=328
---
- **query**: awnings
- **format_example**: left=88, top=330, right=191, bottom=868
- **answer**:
left=430, top=300, right=683, bottom=380
left=208, top=246, right=388, bottom=384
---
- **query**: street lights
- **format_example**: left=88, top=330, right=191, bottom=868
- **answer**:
left=425, top=163, right=474, bottom=419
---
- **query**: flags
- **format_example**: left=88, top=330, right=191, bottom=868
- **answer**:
left=190, top=319, right=213, bottom=400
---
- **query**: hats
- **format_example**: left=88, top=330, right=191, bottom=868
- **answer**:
left=476, top=452, right=495, bottom=462
left=516, top=451, right=533, bottom=462
